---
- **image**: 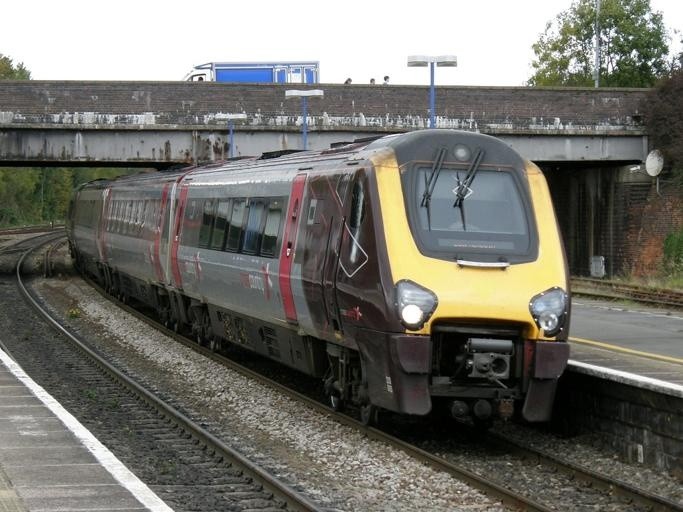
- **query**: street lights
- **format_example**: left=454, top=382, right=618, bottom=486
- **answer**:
left=406, top=53, right=459, bottom=128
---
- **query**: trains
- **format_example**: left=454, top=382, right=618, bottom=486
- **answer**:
left=61, top=133, right=572, bottom=429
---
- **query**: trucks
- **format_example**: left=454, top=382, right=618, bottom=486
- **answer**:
left=182, top=59, right=320, bottom=90
